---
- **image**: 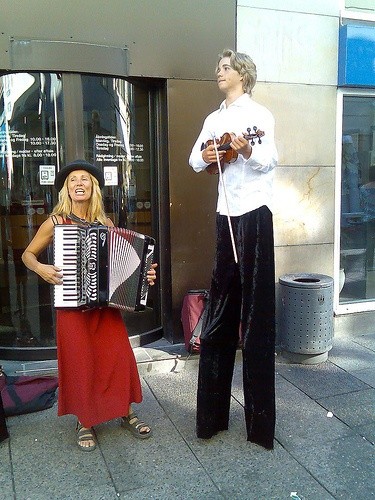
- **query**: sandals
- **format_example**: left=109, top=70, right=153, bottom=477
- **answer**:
left=76, top=420, right=96, bottom=452
left=121, top=414, right=152, bottom=438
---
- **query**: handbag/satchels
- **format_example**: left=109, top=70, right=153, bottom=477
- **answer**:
left=184, top=288, right=242, bottom=354
left=0, top=368, right=59, bottom=416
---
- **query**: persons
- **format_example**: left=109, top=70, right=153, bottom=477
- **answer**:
left=21, top=159, right=158, bottom=452
left=187, top=49, right=278, bottom=449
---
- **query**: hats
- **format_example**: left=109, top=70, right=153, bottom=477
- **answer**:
left=55, top=160, right=105, bottom=189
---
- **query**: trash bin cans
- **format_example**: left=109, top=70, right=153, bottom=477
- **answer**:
left=278, top=273, right=334, bottom=364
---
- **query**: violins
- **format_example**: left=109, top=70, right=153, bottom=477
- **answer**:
left=201, top=126, right=266, bottom=175
